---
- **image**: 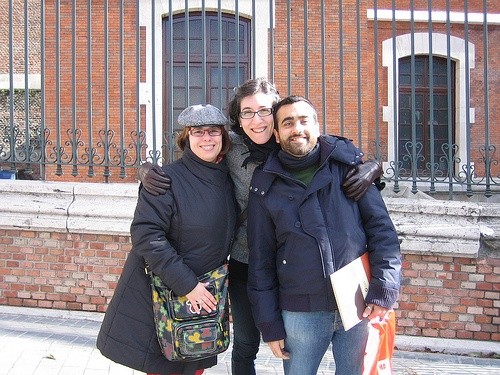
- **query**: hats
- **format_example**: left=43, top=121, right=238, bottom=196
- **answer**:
left=177, top=104, right=229, bottom=126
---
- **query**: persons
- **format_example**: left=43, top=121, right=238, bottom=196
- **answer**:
left=246, top=95, right=402, bottom=375
left=135, top=76, right=385, bottom=375
left=129, top=104, right=239, bottom=375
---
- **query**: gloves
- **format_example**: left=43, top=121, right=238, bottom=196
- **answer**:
left=343, top=159, right=385, bottom=201
left=135, top=162, right=171, bottom=195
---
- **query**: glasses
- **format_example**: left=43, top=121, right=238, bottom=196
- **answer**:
left=188, top=126, right=223, bottom=137
left=238, top=108, right=274, bottom=119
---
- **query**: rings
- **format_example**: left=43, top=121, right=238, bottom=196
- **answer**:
left=367, top=305, right=373, bottom=311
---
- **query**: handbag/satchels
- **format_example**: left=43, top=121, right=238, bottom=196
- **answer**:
left=151, top=264, right=230, bottom=361
left=361, top=307, right=396, bottom=375
